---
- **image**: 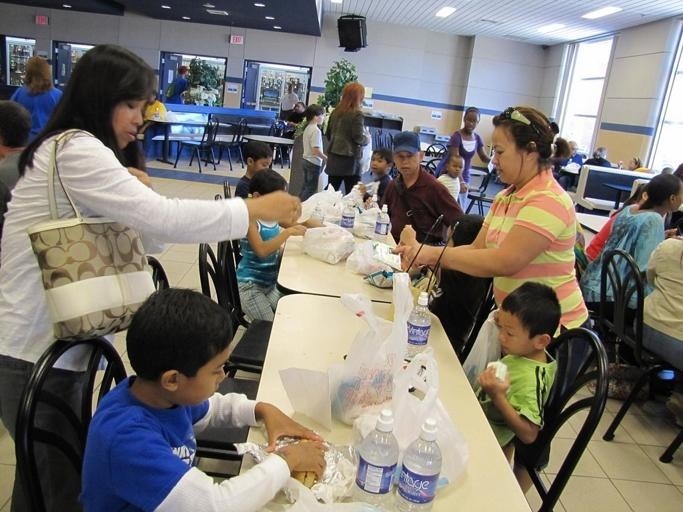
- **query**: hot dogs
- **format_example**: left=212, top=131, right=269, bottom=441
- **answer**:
left=293, top=439, right=320, bottom=488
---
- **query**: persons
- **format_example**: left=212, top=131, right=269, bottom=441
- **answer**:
left=1, top=57, right=62, bottom=208
left=0, top=44, right=303, bottom=511
left=554, top=138, right=610, bottom=191
left=479, top=281, right=562, bottom=448
left=138, top=66, right=189, bottom=157
left=391, top=105, right=593, bottom=347
left=80, top=285, right=330, bottom=511
left=281, top=85, right=304, bottom=126
left=579, top=158, right=683, bottom=372
left=302, top=82, right=369, bottom=202
left=359, top=107, right=492, bottom=288
left=235, top=141, right=305, bottom=321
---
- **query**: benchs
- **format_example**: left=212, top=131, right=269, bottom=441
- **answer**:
left=555, top=151, right=663, bottom=248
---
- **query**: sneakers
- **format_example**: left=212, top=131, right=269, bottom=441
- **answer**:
left=665, top=391, right=683, bottom=427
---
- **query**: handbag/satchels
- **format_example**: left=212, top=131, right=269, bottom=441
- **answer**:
left=587, top=336, right=651, bottom=401
left=26, top=128, right=157, bottom=343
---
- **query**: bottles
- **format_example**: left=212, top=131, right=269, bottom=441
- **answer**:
left=152, top=106, right=160, bottom=121
left=405, top=290, right=431, bottom=367
left=373, top=204, right=391, bottom=245
left=354, top=408, right=398, bottom=509
left=393, top=417, right=442, bottom=510
left=399, top=224, right=415, bottom=246
left=339, top=199, right=355, bottom=233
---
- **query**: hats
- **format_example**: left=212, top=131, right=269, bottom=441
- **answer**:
left=394, top=132, right=421, bottom=154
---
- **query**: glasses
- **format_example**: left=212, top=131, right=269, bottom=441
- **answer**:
left=502, top=107, right=544, bottom=138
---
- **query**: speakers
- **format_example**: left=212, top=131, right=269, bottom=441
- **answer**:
left=337, top=15, right=368, bottom=52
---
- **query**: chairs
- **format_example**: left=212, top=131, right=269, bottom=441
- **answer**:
left=521, top=328, right=610, bottom=512
left=11, top=334, right=130, bottom=511
left=142, top=251, right=261, bottom=461
left=370, top=127, right=496, bottom=175
left=146, top=114, right=297, bottom=174
left=600, top=248, right=683, bottom=463
